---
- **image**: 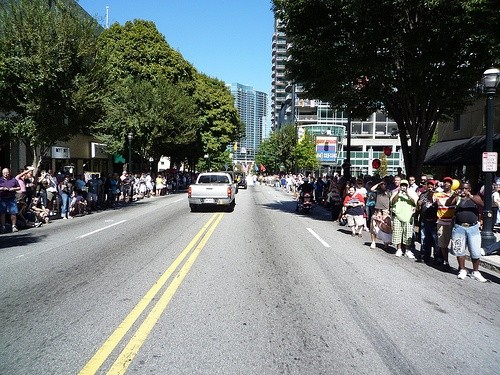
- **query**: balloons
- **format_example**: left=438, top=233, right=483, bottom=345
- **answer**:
left=451, top=179, right=460, bottom=190
left=384, top=146, right=391, bottom=156
left=372, top=159, right=381, bottom=169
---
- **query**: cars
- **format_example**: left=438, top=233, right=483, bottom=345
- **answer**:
left=224, top=170, right=247, bottom=193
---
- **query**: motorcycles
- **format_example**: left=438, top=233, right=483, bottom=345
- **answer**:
left=295, top=186, right=315, bottom=213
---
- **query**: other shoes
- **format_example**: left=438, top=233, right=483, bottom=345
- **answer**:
left=370, top=242, right=376, bottom=249
left=26, top=221, right=35, bottom=227
left=481, top=247, right=489, bottom=256
left=68, top=215, right=73, bottom=219
left=63, top=216, right=67, bottom=219
left=75, top=213, right=84, bottom=217
left=36, top=222, right=42, bottom=227
left=1, top=224, right=6, bottom=234
left=84, top=211, right=88, bottom=214
left=12, top=225, right=18, bottom=232
left=358, top=234, right=362, bottom=238
left=351, top=233, right=355, bottom=237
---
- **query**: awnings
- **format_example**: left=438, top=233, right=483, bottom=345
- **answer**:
left=114, top=149, right=147, bottom=164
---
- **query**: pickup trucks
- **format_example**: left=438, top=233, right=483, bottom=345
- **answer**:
left=187, top=172, right=237, bottom=212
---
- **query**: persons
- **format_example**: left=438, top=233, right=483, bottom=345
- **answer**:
left=263, top=167, right=500, bottom=256
left=0, top=165, right=199, bottom=232
left=322, top=127, right=331, bottom=135
left=258, top=174, right=263, bottom=185
left=445, top=181, right=487, bottom=282
left=252, top=174, right=257, bottom=185
left=415, top=180, right=438, bottom=265
left=389, top=180, right=419, bottom=259
left=428, top=177, right=460, bottom=271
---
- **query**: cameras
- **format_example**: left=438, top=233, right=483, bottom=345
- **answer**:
left=458, top=190, right=466, bottom=197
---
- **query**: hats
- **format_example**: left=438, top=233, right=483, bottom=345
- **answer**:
left=443, top=177, right=453, bottom=183
left=426, top=174, right=433, bottom=179
left=399, top=179, right=409, bottom=188
left=427, top=180, right=436, bottom=188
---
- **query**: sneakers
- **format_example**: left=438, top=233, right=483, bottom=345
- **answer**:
left=396, top=250, right=402, bottom=257
left=404, top=251, right=415, bottom=258
left=457, top=270, right=467, bottom=279
left=471, top=271, right=487, bottom=283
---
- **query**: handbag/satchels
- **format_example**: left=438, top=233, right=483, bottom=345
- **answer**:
left=339, top=214, right=347, bottom=226
left=378, top=214, right=392, bottom=234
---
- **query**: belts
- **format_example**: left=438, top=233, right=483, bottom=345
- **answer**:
left=455, top=221, right=477, bottom=227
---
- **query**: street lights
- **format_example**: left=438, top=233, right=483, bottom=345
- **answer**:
left=127, top=131, right=134, bottom=202
left=480, top=67, right=500, bottom=250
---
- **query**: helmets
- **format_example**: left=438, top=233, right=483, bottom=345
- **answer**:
left=304, top=178, right=309, bottom=182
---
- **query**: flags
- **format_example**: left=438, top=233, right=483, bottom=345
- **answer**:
left=255, top=164, right=259, bottom=171
left=248, top=162, right=252, bottom=169
left=261, top=164, right=266, bottom=172
left=225, top=162, right=238, bottom=172
left=242, top=164, right=246, bottom=173
